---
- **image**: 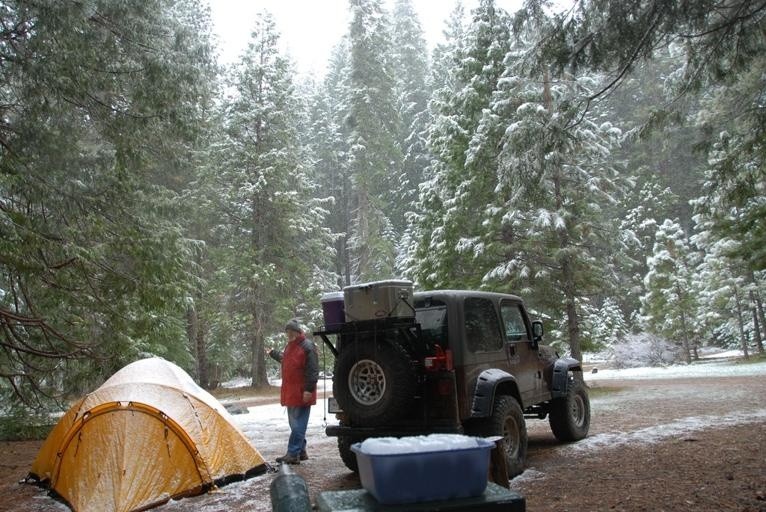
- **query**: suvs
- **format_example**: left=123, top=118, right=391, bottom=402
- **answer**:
left=313, top=289, right=591, bottom=480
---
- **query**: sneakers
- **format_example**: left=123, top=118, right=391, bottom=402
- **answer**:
left=276, top=451, right=309, bottom=465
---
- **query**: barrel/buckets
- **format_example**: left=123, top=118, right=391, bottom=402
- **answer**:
left=320, top=292, right=345, bottom=332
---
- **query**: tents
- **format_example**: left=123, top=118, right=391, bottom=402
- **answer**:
left=18, top=356, right=274, bottom=512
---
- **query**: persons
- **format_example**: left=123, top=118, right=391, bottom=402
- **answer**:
left=262, top=320, right=319, bottom=464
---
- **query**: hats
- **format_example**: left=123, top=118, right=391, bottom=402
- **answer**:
left=284, top=317, right=303, bottom=334
